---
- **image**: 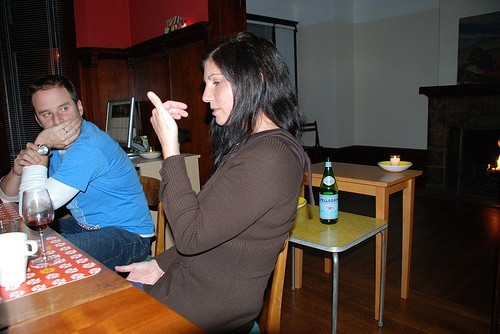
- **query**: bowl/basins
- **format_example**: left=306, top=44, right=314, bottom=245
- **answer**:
left=378, top=160, right=413, bottom=172
left=139, top=152, right=161, bottom=159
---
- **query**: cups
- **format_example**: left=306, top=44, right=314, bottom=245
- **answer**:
left=390, top=155, right=401, bottom=166
left=0, top=232, right=38, bottom=286
left=0, top=220, right=18, bottom=235
left=147, top=146, right=155, bottom=153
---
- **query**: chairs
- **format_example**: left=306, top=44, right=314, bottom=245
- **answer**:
left=138, top=173, right=165, bottom=257
left=255, top=239, right=285, bottom=334
left=300, top=120, right=326, bottom=163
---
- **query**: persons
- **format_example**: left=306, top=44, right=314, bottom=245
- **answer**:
left=0, top=75, right=155, bottom=272
left=115, top=32, right=314, bottom=334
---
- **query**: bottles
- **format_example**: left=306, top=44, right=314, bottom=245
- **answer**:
left=142, top=136, right=148, bottom=149
left=319, top=156, right=339, bottom=224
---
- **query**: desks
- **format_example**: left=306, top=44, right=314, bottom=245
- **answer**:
left=290, top=200, right=389, bottom=334
left=129, top=151, right=202, bottom=196
left=0, top=198, right=206, bottom=334
left=294, top=161, right=422, bottom=320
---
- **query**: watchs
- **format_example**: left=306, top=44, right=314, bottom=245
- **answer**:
left=36, top=144, right=51, bottom=156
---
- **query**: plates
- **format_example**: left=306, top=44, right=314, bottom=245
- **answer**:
left=297, top=197, right=307, bottom=210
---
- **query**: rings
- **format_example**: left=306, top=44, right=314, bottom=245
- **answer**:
left=63, top=128, right=69, bottom=133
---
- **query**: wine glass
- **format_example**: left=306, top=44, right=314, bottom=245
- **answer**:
left=22, top=187, right=59, bottom=266
